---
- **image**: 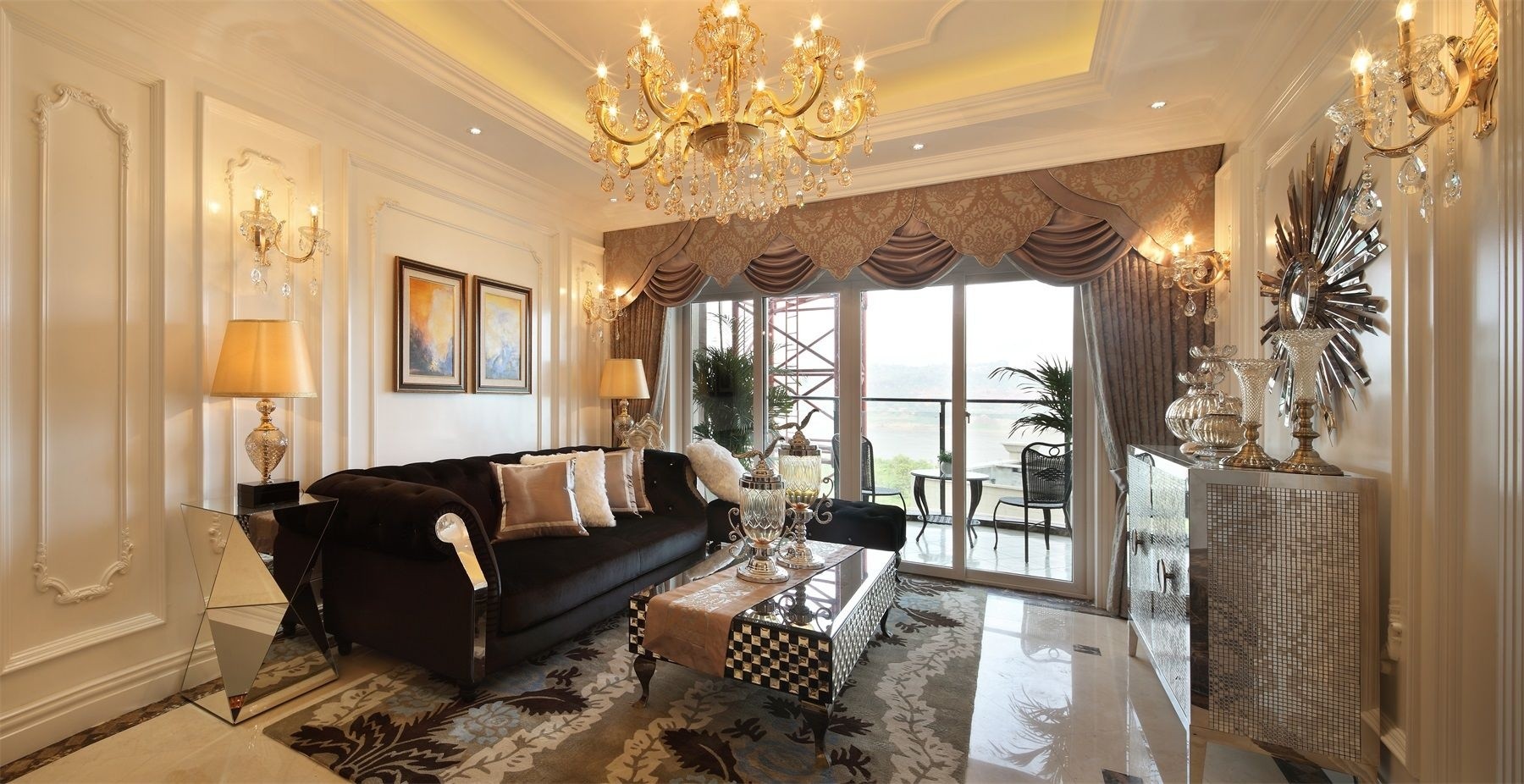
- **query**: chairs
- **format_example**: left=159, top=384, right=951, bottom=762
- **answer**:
left=830, top=434, right=907, bottom=518
left=991, top=441, right=1075, bottom=562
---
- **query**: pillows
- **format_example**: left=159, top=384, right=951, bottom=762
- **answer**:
left=487, top=458, right=591, bottom=545
left=629, top=447, right=656, bottom=515
left=571, top=448, right=643, bottom=517
left=519, top=449, right=615, bottom=527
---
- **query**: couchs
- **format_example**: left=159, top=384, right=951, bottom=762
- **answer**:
left=706, top=491, right=908, bottom=583
left=310, top=444, right=712, bottom=708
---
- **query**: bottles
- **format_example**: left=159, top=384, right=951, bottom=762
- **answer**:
left=1165, top=344, right=1246, bottom=462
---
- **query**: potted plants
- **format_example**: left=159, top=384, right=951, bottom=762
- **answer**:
left=936, top=449, right=952, bottom=474
left=691, top=342, right=799, bottom=480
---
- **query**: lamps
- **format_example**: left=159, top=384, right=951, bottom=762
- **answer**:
left=273, top=178, right=334, bottom=299
left=1328, top=1, right=1499, bottom=217
left=600, top=356, right=651, bottom=445
left=213, top=318, right=317, bottom=504
left=240, top=182, right=282, bottom=292
left=583, top=0, right=883, bottom=227
left=1160, top=228, right=1231, bottom=325
left=579, top=275, right=629, bottom=351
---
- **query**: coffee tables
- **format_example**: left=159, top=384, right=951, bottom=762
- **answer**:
left=626, top=534, right=898, bottom=770
left=177, top=489, right=341, bottom=725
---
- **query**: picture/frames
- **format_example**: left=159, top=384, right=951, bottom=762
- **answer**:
left=393, top=255, right=470, bottom=395
left=471, top=274, right=532, bottom=395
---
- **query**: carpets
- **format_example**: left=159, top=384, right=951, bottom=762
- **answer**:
left=257, top=575, right=990, bottom=784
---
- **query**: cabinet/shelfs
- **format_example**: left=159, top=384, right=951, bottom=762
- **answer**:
left=1124, top=442, right=1382, bottom=783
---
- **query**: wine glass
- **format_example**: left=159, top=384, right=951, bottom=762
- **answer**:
left=773, top=408, right=834, bottom=571
left=1218, top=326, right=1347, bottom=476
left=728, top=436, right=799, bottom=584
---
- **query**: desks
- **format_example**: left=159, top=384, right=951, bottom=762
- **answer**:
left=908, top=468, right=991, bottom=549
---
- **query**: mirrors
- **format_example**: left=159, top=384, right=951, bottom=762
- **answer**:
left=224, top=155, right=289, bottom=506
